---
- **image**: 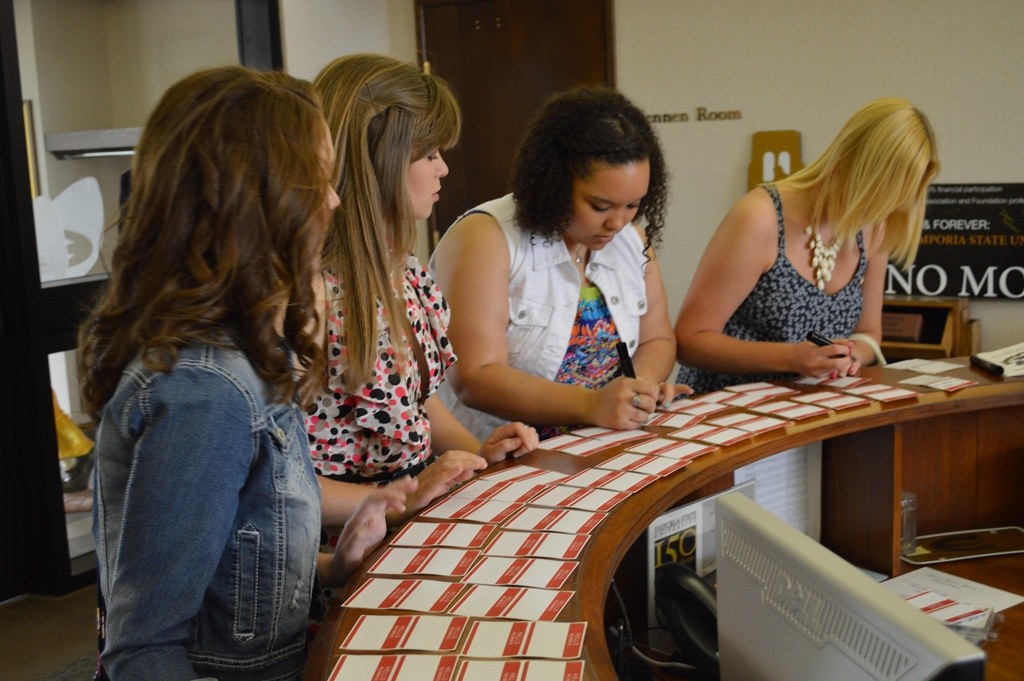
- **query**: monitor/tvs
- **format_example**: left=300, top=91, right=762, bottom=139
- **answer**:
left=718, top=492, right=986, bottom=681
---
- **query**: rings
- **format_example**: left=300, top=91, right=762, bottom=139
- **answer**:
left=523, top=425, right=530, bottom=430
left=632, top=393, right=640, bottom=408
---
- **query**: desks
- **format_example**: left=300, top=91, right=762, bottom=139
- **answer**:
left=308, top=349, right=1023, bottom=681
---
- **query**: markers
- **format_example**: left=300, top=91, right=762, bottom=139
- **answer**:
left=617, top=341, right=636, bottom=378
left=806, top=330, right=857, bottom=363
left=968, top=354, right=1004, bottom=377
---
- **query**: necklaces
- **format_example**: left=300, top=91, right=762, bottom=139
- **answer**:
left=805, top=220, right=845, bottom=291
left=566, top=240, right=585, bottom=265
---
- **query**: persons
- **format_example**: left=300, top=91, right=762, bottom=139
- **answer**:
left=75, top=66, right=420, bottom=681
left=288, top=54, right=541, bottom=525
left=675, top=98, right=938, bottom=400
left=425, top=87, right=695, bottom=431
